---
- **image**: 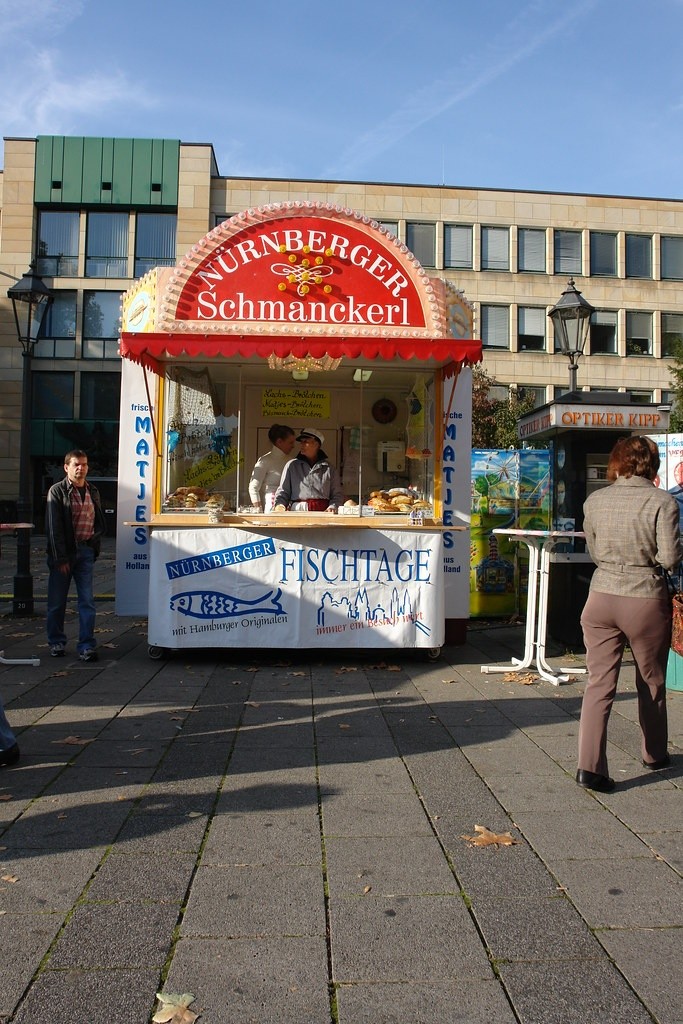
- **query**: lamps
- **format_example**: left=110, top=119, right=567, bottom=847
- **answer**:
left=352, top=368, right=373, bottom=384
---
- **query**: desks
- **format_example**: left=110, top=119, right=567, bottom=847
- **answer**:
left=480, top=529, right=596, bottom=685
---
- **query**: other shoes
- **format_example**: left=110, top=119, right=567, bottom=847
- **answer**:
left=50, top=643, right=66, bottom=656
left=80, top=649, right=97, bottom=661
left=0, top=744, right=20, bottom=767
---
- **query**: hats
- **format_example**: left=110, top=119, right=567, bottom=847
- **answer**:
left=296, top=427, right=325, bottom=444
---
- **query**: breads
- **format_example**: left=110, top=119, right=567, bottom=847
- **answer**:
left=344, top=499, right=357, bottom=506
left=165, top=485, right=224, bottom=512
left=367, top=490, right=432, bottom=512
left=275, top=504, right=286, bottom=511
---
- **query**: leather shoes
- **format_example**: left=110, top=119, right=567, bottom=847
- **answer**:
left=643, top=756, right=671, bottom=770
left=576, top=769, right=616, bottom=793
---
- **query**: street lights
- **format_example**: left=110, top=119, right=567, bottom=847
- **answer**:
left=5, top=260, right=56, bottom=620
left=547, top=274, right=596, bottom=553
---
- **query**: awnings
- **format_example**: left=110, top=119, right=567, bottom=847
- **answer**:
left=121, top=331, right=483, bottom=377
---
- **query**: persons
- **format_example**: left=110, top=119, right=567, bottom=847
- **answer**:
left=576, top=436, right=683, bottom=793
left=248, top=424, right=295, bottom=513
left=1, top=695, right=20, bottom=767
left=46, top=451, right=103, bottom=661
left=274, top=427, right=343, bottom=514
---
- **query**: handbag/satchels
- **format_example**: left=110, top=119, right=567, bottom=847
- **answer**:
left=663, top=569, right=683, bottom=657
